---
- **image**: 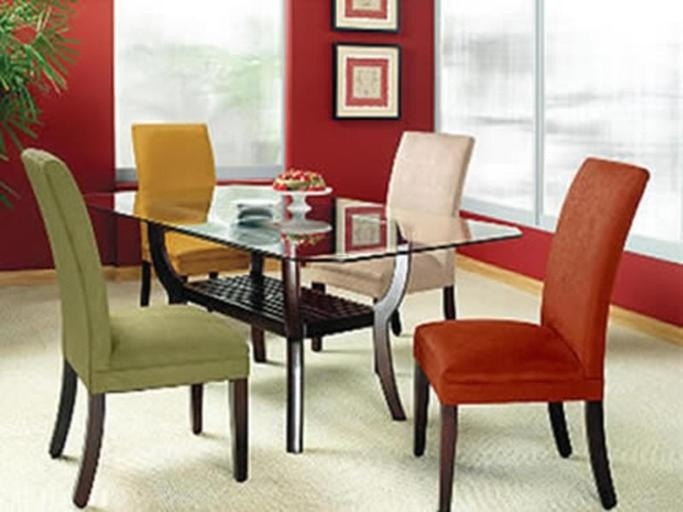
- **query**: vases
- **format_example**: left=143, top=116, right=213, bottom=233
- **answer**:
left=275, top=187, right=332, bottom=237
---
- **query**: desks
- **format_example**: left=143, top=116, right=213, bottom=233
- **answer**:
left=83, top=186, right=521, bottom=452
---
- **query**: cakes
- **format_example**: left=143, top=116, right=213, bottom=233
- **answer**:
left=284, top=233, right=330, bottom=247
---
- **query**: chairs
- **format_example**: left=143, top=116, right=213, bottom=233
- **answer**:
left=19, top=148, right=249, bottom=512
left=131, top=123, right=255, bottom=305
left=308, top=132, right=474, bottom=373
left=413, top=158, right=650, bottom=511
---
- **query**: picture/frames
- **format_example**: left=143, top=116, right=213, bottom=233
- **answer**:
left=333, top=44, right=400, bottom=121
left=334, top=200, right=395, bottom=257
left=332, top=0, right=400, bottom=33
